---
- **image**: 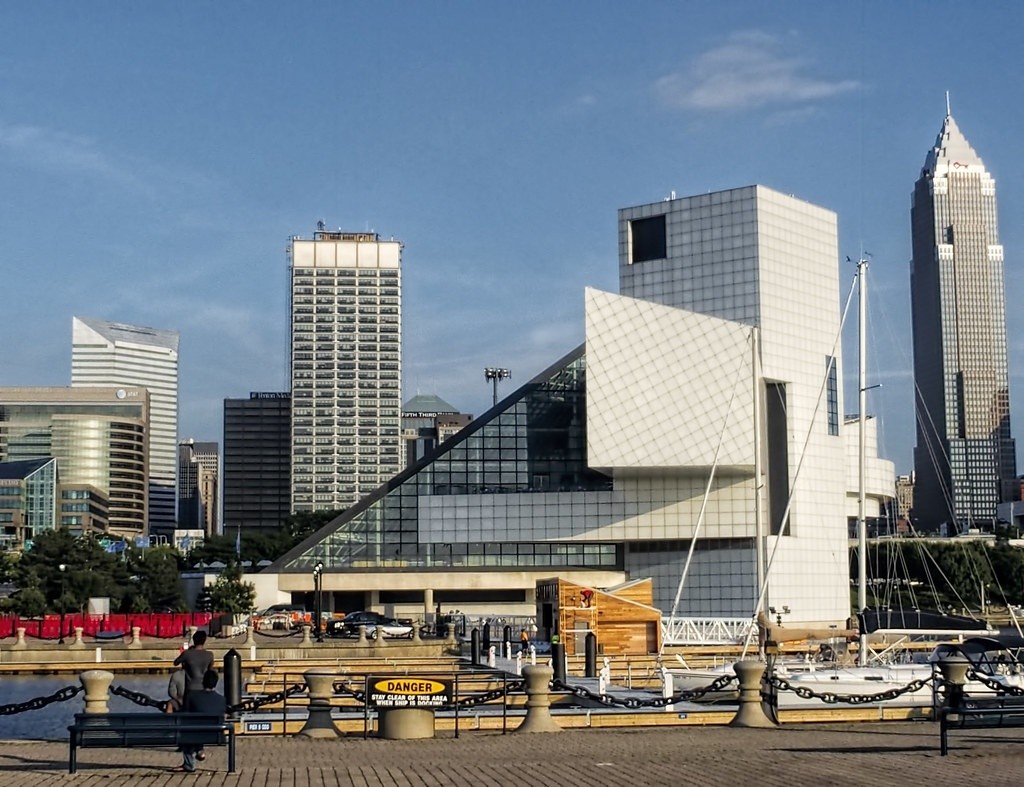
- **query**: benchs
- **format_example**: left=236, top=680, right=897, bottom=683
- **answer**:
left=939, top=695, right=1024, bottom=756
left=66, top=710, right=236, bottom=774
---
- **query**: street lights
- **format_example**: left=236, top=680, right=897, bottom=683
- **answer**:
left=312, top=561, right=322, bottom=635
left=58, top=563, right=67, bottom=645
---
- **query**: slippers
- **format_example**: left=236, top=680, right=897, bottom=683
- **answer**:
left=195, top=754, right=205, bottom=761
left=173, top=765, right=197, bottom=772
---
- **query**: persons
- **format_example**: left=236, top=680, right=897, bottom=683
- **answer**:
left=177, top=670, right=227, bottom=771
left=520, top=629, right=531, bottom=656
left=173, top=630, right=215, bottom=760
left=552, top=632, right=561, bottom=645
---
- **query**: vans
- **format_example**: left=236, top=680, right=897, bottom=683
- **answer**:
left=246, top=604, right=305, bottom=630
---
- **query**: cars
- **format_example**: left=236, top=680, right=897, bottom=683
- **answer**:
left=359, top=617, right=414, bottom=639
left=327, top=611, right=389, bottom=638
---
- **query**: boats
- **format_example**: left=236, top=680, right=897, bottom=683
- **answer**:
left=655, top=258, right=1024, bottom=703
